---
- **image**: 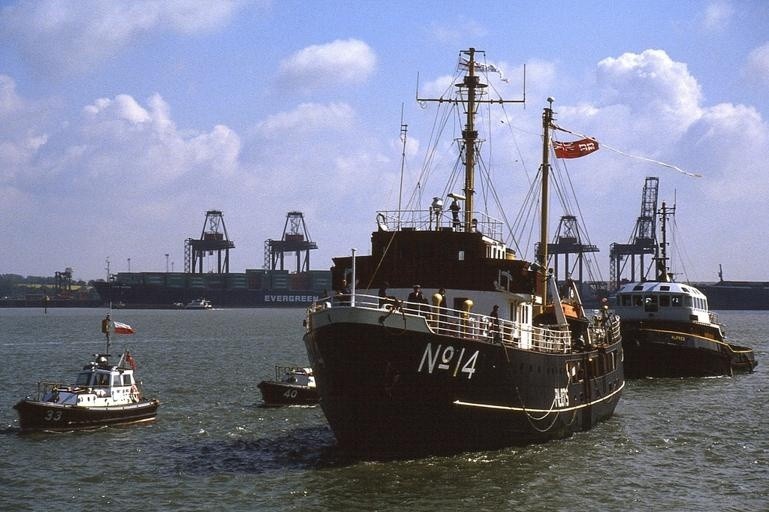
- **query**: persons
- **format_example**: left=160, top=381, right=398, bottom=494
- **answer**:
left=439, top=287, right=449, bottom=333
left=378, top=280, right=390, bottom=309
left=335, top=277, right=352, bottom=307
left=131, top=384, right=138, bottom=398
left=489, top=305, right=500, bottom=344
left=450, top=197, right=461, bottom=221
left=406, top=284, right=424, bottom=317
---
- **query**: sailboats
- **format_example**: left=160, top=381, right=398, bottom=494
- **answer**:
left=606, top=197, right=757, bottom=376
left=300, top=47, right=625, bottom=458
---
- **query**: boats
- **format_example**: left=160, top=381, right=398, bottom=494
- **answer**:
left=12, top=302, right=160, bottom=432
left=185, top=297, right=212, bottom=309
left=257, top=366, right=319, bottom=408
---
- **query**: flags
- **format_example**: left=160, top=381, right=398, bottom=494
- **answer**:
left=113, top=319, right=137, bottom=336
left=125, top=350, right=138, bottom=373
left=552, top=135, right=600, bottom=158
